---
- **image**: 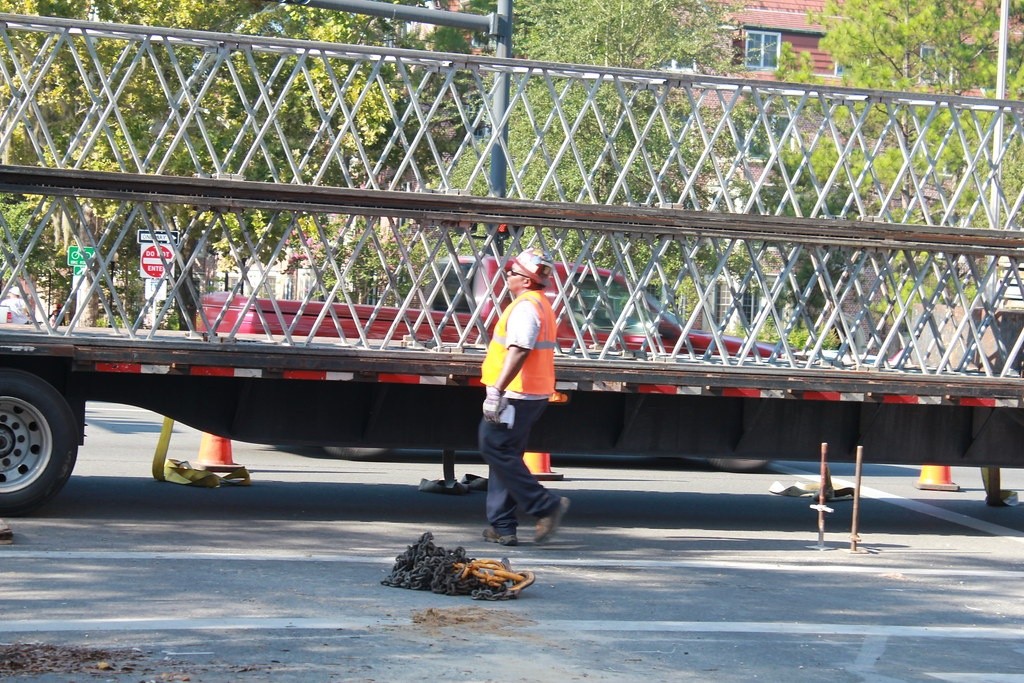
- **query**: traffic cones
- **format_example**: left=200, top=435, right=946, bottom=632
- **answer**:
left=192, top=430, right=247, bottom=474
left=912, top=464, right=960, bottom=492
left=521, top=452, right=564, bottom=481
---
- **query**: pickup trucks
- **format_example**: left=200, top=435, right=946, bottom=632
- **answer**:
left=195, top=254, right=794, bottom=473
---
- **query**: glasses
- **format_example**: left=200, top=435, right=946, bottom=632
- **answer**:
left=508, top=267, right=529, bottom=279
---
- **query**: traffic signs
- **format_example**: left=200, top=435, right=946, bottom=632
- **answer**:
left=137, top=228, right=179, bottom=245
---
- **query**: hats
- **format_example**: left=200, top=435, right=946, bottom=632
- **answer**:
left=510, top=246, right=555, bottom=290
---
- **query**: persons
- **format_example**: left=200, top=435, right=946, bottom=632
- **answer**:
left=1, top=286, right=30, bottom=325
left=477, top=247, right=570, bottom=546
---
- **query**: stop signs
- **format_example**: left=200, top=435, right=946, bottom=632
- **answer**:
left=140, top=244, right=175, bottom=280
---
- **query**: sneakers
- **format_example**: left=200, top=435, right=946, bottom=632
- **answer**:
left=533, top=496, right=570, bottom=544
left=480, top=526, right=517, bottom=546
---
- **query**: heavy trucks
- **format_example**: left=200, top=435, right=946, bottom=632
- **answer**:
left=0, top=0, right=1023, bottom=525
